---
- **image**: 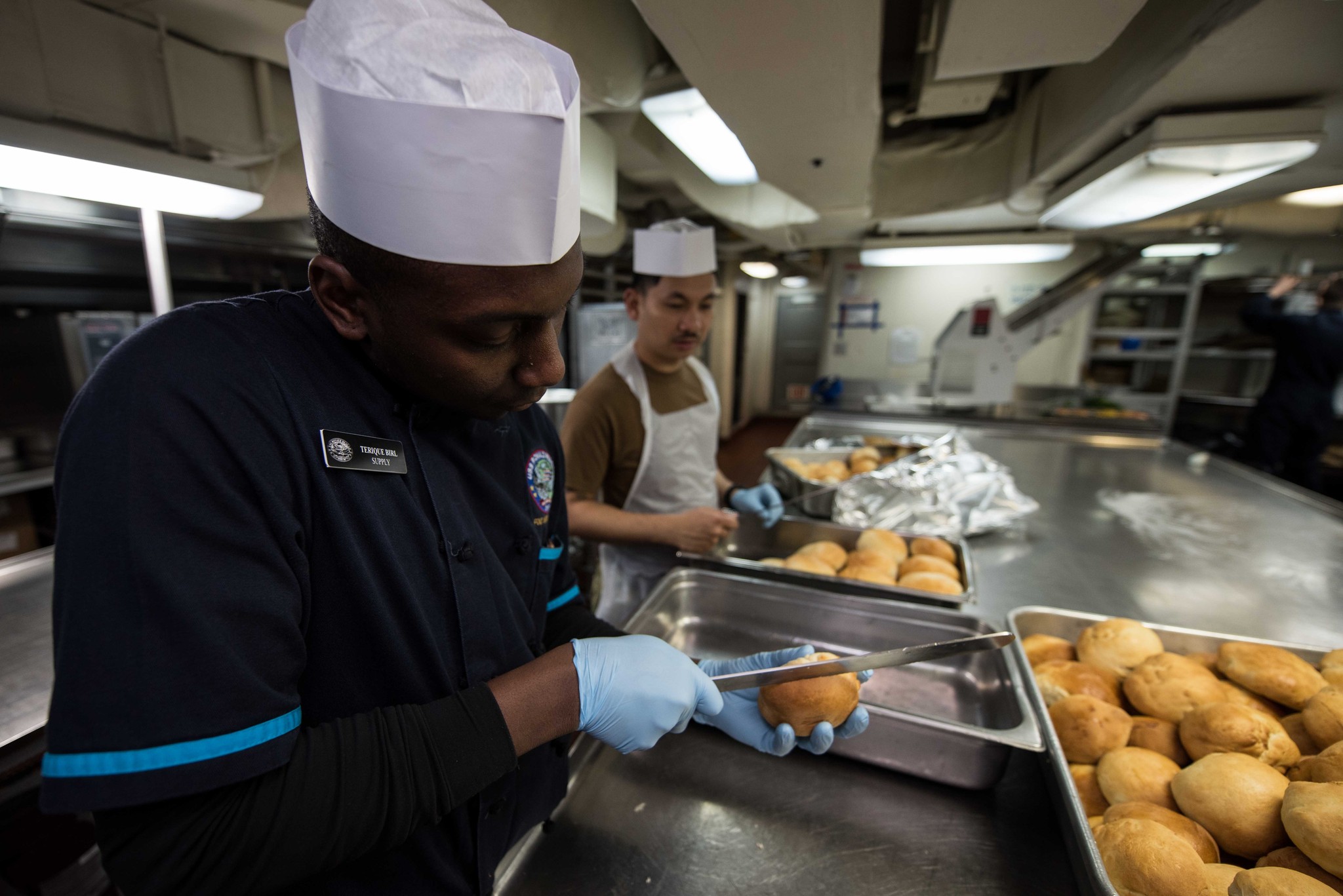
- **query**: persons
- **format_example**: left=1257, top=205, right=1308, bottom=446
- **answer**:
left=42, top=0, right=874, bottom=896
left=559, top=216, right=785, bottom=630
left=1211, top=270, right=1343, bottom=489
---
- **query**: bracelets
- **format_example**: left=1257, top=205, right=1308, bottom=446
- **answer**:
left=724, top=483, right=744, bottom=510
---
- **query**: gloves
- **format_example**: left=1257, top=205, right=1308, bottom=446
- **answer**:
left=569, top=632, right=722, bottom=756
left=730, top=482, right=785, bottom=528
left=690, top=644, right=874, bottom=757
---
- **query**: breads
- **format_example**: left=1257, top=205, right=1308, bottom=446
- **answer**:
left=781, top=436, right=911, bottom=482
left=757, top=652, right=861, bottom=737
left=1023, top=617, right=1343, bottom=896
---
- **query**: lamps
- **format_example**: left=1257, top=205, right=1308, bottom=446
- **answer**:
left=858, top=229, right=1074, bottom=267
left=640, top=62, right=761, bottom=185
left=0, top=115, right=264, bottom=221
left=1035, top=109, right=1327, bottom=235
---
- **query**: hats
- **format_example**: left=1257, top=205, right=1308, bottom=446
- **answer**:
left=285, top=2, right=582, bottom=267
left=633, top=218, right=719, bottom=278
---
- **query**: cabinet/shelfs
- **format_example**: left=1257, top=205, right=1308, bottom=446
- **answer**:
left=1075, top=252, right=1207, bottom=439
